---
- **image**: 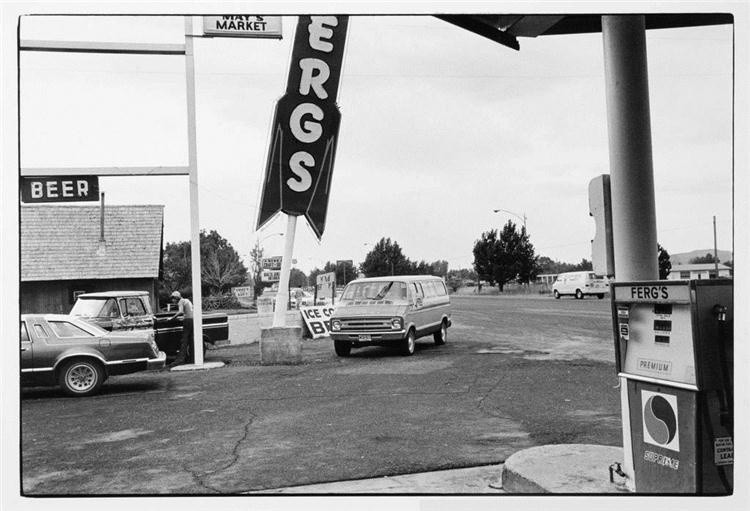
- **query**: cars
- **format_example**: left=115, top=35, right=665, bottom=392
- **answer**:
left=20, top=313, right=167, bottom=397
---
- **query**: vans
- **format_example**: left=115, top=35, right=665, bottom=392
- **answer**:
left=552, top=271, right=611, bottom=299
left=329, top=275, right=452, bottom=356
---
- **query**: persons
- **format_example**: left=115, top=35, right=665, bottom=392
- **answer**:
left=294, top=284, right=306, bottom=310
left=167, top=290, right=193, bottom=367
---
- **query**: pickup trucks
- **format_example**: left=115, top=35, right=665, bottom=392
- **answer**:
left=69, top=290, right=229, bottom=364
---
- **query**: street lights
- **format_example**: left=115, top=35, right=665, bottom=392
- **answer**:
left=493, top=209, right=527, bottom=237
left=252, top=232, right=283, bottom=269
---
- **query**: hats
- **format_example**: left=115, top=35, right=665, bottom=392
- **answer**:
left=169, top=291, right=182, bottom=298
